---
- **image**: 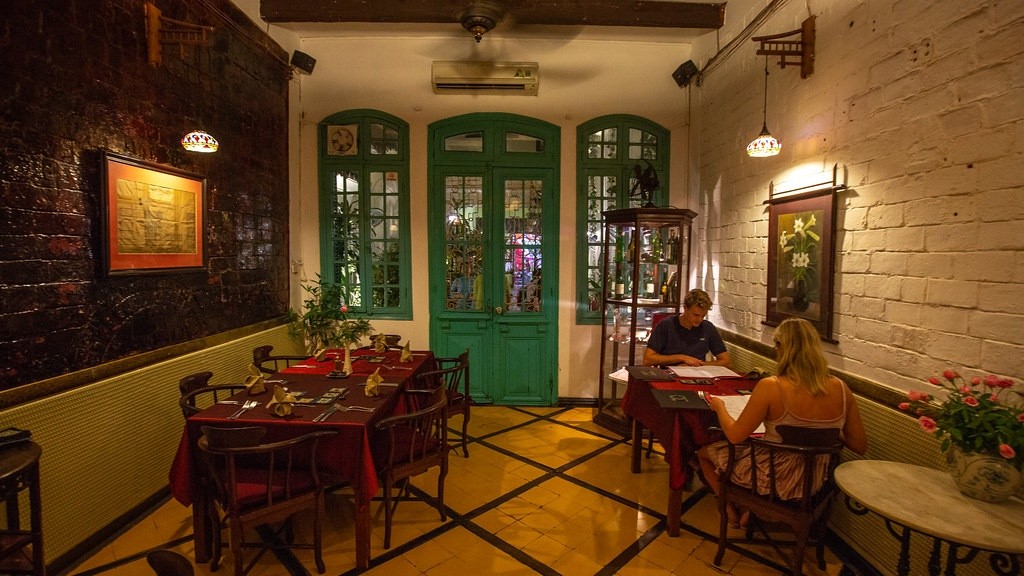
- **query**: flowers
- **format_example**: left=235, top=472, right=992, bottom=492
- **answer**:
left=898, top=367, right=1024, bottom=468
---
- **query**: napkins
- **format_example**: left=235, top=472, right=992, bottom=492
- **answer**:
left=400, top=341, right=413, bottom=363
left=313, top=338, right=327, bottom=362
left=243, top=363, right=265, bottom=395
left=364, top=367, right=383, bottom=397
left=371, top=333, right=387, bottom=353
left=266, top=385, right=296, bottom=416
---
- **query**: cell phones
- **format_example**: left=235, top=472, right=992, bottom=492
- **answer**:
left=736, top=389, right=751, bottom=394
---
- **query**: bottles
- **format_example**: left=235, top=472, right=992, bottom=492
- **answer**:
left=628, top=231, right=637, bottom=262
left=614, top=268, right=624, bottom=300
left=654, top=234, right=665, bottom=262
left=665, top=230, right=678, bottom=264
left=615, top=229, right=624, bottom=263
left=660, top=271, right=668, bottom=301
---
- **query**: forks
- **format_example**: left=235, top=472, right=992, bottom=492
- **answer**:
left=697, top=390, right=710, bottom=407
left=382, top=365, right=412, bottom=371
left=227, top=400, right=257, bottom=419
left=334, top=403, right=376, bottom=413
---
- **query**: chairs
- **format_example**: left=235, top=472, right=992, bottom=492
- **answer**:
left=178, top=332, right=470, bottom=573
left=707, top=426, right=842, bottom=576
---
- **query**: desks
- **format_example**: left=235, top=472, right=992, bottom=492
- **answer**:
left=629, top=367, right=769, bottom=538
left=833, top=460, right=1024, bottom=576
left=0, top=430, right=45, bottom=576
left=187, top=348, right=433, bottom=570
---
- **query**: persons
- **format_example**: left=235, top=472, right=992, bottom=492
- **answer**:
left=644, top=289, right=733, bottom=463
left=697, top=319, right=868, bottom=530
left=449, top=234, right=540, bottom=312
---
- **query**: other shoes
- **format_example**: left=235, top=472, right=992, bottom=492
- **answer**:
left=696, top=468, right=717, bottom=496
left=716, top=502, right=751, bottom=530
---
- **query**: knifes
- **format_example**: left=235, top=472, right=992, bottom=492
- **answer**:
left=313, top=407, right=338, bottom=423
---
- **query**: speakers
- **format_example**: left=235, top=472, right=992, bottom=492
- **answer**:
left=290, top=51, right=316, bottom=73
left=672, top=60, right=698, bottom=87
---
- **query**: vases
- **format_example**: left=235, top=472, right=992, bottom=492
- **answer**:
left=942, top=440, right=1020, bottom=504
left=343, top=344, right=353, bottom=376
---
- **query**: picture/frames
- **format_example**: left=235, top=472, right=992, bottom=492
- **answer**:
left=761, top=163, right=849, bottom=347
left=98, top=148, right=208, bottom=280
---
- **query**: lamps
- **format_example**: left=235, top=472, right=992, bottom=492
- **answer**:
left=290, top=49, right=317, bottom=76
left=672, top=59, right=702, bottom=88
left=181, top=42, right=219, bottom=152
left=747, top=56, right=782, bottom=157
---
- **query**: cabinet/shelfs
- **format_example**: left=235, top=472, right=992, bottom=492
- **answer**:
left=592, top=207, right=699, bottom=439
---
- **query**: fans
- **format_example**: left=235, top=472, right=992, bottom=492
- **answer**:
left=627, top=158, right=660, bottom=208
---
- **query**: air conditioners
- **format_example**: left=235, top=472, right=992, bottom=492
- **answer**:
left=430, top=61, right=538, bottom=95
